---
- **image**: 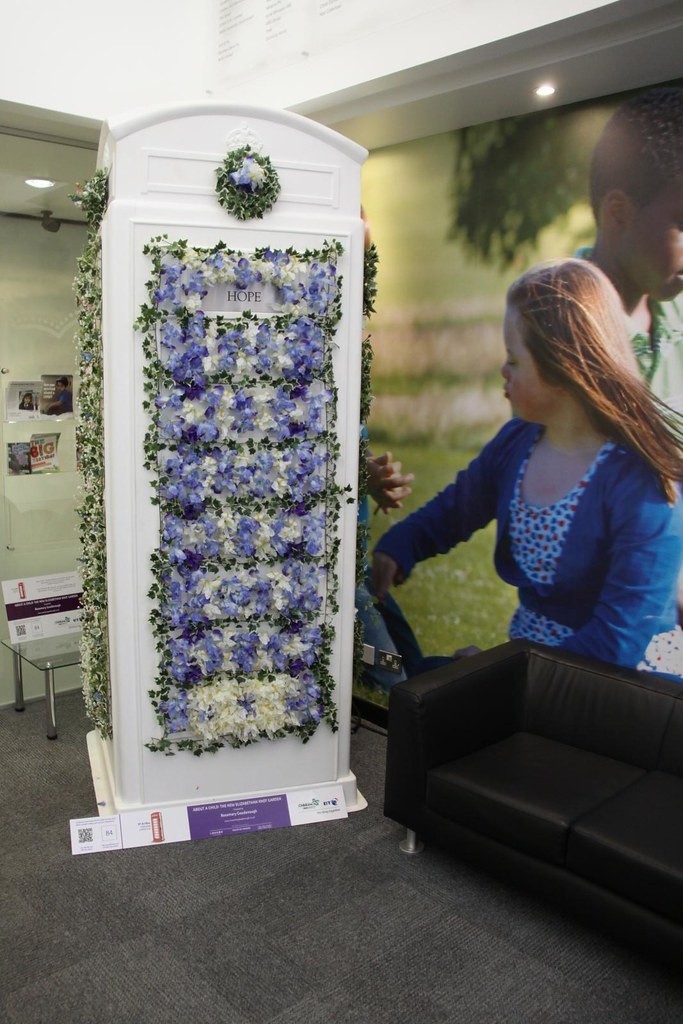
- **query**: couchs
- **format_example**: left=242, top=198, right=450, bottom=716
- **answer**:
left=380, top=634, right=683, bottom=972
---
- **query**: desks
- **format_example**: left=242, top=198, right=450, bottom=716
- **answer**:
left=1, top=629, right=86, bottom=740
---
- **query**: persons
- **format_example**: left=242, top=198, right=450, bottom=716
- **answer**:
left=572, top=86, right=683, bottom=629
left=371, top=257, right=682, bottom=693
left=43, top=376, right=74, bottom=416
left=19, top=393, right=34, bottom=411
left=354, top=445, right=415, bottom=693
